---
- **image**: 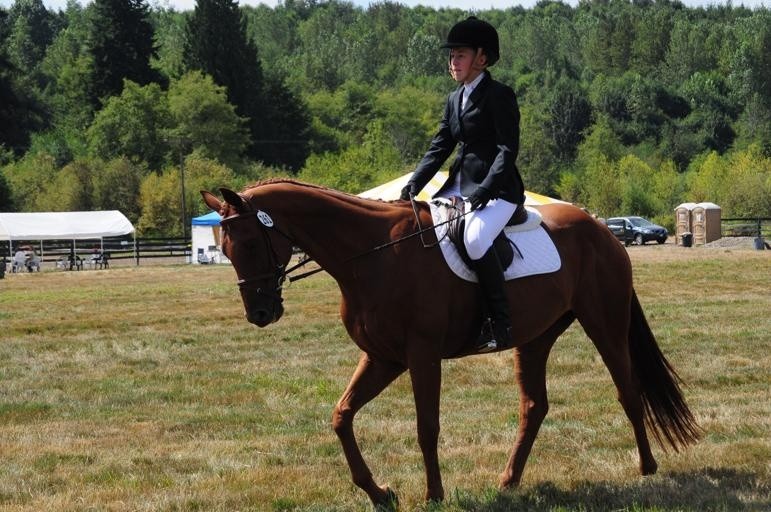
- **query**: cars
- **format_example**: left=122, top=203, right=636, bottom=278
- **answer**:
left=604, top=215, right=669, bottom=247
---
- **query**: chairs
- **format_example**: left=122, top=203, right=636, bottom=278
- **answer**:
left=6, top=255, right=42, bottom=273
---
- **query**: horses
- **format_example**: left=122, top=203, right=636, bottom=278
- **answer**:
left=198, top=178, right=708, bottom=512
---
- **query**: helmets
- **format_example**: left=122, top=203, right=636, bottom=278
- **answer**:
left=439, top=16, right=499, bottom=66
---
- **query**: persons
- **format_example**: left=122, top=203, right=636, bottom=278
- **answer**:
left=26, top=245, right=39, bottom=272
left=67, top=252, right=80, bottom=271
left=9, top=247, right=26, bottom=273
left=399, top=15, right=526, bottom=352
left=90, top=250, right=103, bottom=269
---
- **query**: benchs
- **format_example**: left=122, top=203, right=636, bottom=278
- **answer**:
left=55, top=259, right=111, bottom=271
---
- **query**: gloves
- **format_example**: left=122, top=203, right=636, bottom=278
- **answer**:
left=468, top=187, right=489, bottom=211
left=401, top=180, right=418, bottom=200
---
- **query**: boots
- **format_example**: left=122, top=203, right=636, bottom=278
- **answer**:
left=470, top=245, right=512, bottom=346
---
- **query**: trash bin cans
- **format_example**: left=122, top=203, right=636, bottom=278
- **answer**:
left=754, top=238, right=765, bottom=250
left=682, top=232, right=693, bottom=247
